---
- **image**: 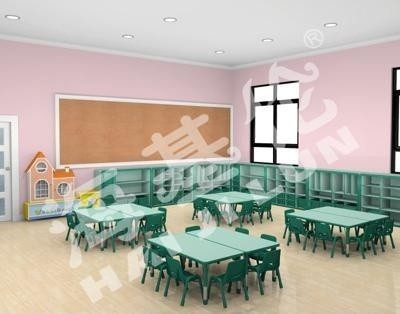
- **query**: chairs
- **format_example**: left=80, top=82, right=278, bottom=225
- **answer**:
left=192, top=190, right=274, bottom=226
left=281, top=205, right=396, bottom=259
left=141, top=225, right=284, bottom=308
left=65, top=202, right=167, bottom=252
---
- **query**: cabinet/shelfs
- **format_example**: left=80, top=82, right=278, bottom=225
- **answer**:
left=93, top=161, right=234, bottom=209
left=234, top=162, right=400, bottom=228
